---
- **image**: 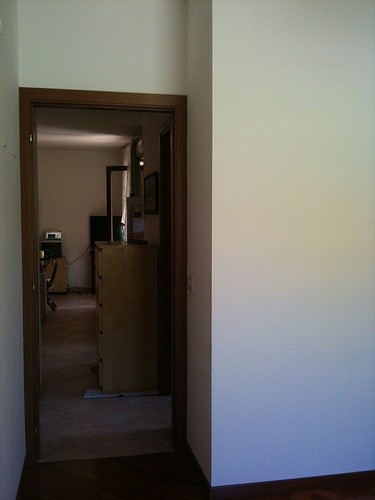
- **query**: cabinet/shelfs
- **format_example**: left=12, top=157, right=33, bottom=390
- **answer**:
left=46, top=257, right=67, bottom=294
left=93, top=241, right=158, bottom=393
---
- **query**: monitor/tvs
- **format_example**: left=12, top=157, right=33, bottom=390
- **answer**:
left=90, top=215, right=121, bottom=249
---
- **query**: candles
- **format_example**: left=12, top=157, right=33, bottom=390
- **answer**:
left=39, top=250, right=44, bottom=258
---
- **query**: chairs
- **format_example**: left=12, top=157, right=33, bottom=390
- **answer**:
left=46, top=260, right=57, bottom=311
